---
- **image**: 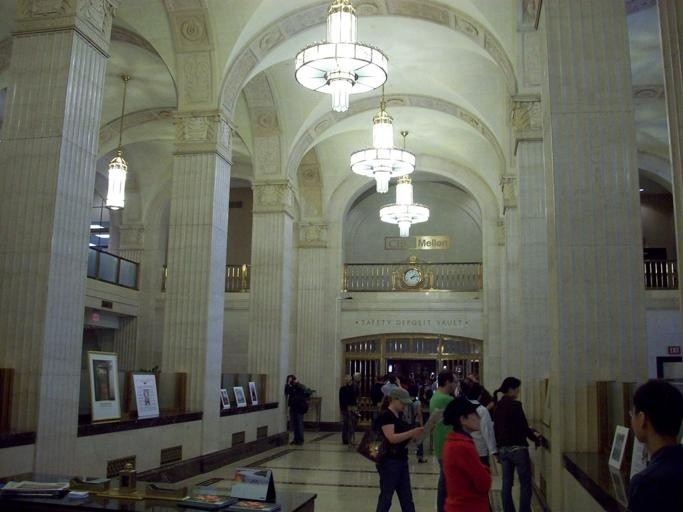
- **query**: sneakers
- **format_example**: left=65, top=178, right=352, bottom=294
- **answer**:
left=418, top=456, right=428, bottom=463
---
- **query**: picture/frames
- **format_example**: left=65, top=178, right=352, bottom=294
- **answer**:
left=248, top=382, right=259, bottom=405
left=609, top=426, right=630, bottom=470
left=233, top=386, right=247, bottom=408
left=87, top=351, right=122, bottom=424
left=220, top=389, right=230, bottom=410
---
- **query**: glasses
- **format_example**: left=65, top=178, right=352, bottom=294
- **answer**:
left=628, top=410, right=638, bottom=417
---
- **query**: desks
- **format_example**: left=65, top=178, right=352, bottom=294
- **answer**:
left=0, top=471, right=317, bottom=512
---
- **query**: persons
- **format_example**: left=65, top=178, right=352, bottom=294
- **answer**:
left=622, top=375, right=682, bottom=510
left=335, top=360, right=542, bottom=511
left=280, top=371, right=310, bottom=445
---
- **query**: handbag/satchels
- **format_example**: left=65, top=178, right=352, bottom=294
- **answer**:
left=356, top=412, right=396, bottom=465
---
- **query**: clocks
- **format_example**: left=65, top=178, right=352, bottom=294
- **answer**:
left=403, top=268, right=422, bottom=287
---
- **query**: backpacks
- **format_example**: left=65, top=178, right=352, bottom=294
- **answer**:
left=401, top=399, right=418, bottom=426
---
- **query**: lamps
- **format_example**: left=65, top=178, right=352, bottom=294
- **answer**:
left=379, top=131, right=430, bottom=238
left=349, top=85, right=416, bottom=193
left=106, top=75, right=131, bottom=211
left=295, top=0, right=389, bottom=112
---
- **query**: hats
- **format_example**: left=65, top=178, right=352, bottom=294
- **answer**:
left=389, top=388, right=413, bottom=405
left=442, top=397, right=480, bottom=426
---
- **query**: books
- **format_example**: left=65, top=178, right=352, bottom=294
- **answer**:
left=2, top=479, right=69, bottom=498
left=177, top=492, right=239, bottom=509
left=228, top=499, right=284, bottom=511
